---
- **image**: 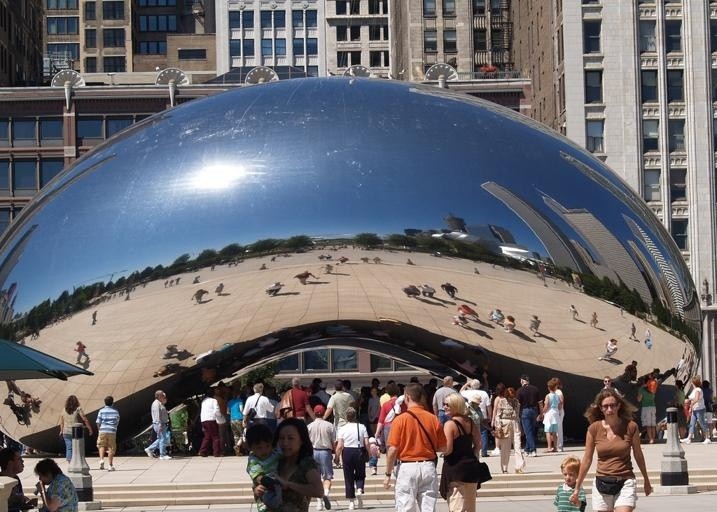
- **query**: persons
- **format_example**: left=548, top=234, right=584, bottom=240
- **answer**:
left=645, top=327, right=652, bottom=347
left=589, top=312, right=599, bottom=327
left=554, top=456, right=586, bottom=511
left=4, top=379, right=41, bottom=426
left=58, top=393, right=93, bottom=465
left=146, top=390, right=172, bottom=460
left=567, top=304, right=579, bottom=322
left=406, top=258, right=415, bottom=266
left=568, top=388, right=654, bottom=511
left=383, top=384, right=447, bottom=512
left=196, top=374, right=489, bottom=457
left=308, top=406, right=370, bottom=509
left=418, top=282, right=437, bottom=297
left=500, top=316, right=517, bottom=335
left=107, top=252, right=383, bottom=305
left=529, top=315, right=541, bottom=337
left=491, top=375, right=565, bottom=472
left=458, top=305, right=482, bottom=325
left=402, top=284, right=420, bottom=297
left=449, top=313, right=470, bottom=329
left=674, top=345, right=701, bottom=384
left=435, top=392, right=483, bottom=512
left=95, top=394, right=121, bottom=473
left=30, top=325, right=41, bottom=342
left=161, top=344, right=183, bottom=359
left=629, top=321, right=640, bottom=343
left=598, top=338, right=619, bottom=363
left=441, top=281, right=460, bottom=300
left=90, top=309, right=99, bottom=327
left=73, top=340, right=91, bottom=365
left=252, top=418, right=325, bottom=512
left=487, top=309, right=505, bottom=325
left=35, top=456, right=79, bottom=511
left=21, top=338, right=26, bottom=346
left=0, top=447, right=32, bottom=511
left=244, top=423, right=324, bottom=511
left=597, top=360, right=715, bottom=443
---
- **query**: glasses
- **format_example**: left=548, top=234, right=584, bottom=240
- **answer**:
left=443, top=402, right=449, bottom=407
left=599, top=403, right=619, bottom=408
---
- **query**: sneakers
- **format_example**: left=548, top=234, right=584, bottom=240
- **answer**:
left=680, top=438, right=691, bottom=444
left=701, top=439, right=711, bottom=444
left=524, top=451, right=537, bottom=457
left=100, top=460, right=115, bottom=471
left=316, top=493, right=331, bottom=511
left=145, top=448, right=172, bottom=459
left=349, top=490, right=362, bottom=510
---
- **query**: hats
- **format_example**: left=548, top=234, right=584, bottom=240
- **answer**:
left=314, top=404, right=324, bottom=416
left=471, top=394, right=481, bottom=404
left=368, top=437, right=376, bottom=444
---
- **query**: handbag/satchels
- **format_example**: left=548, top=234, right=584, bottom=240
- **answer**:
left=494, top=421, right=512, bottom=439
left=247, top=408, right=256, bottom=421
left=595, top=476, right=627, bottom=495
left=357, top=447, right=371, bottom=461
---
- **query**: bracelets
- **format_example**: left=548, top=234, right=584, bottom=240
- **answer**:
left=40, top=490, right=45, bottom=494
left=385, top=470, right=392, bottom=479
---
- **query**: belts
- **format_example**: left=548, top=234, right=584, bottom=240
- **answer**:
left=398, top=460, right=434, bottom=464
